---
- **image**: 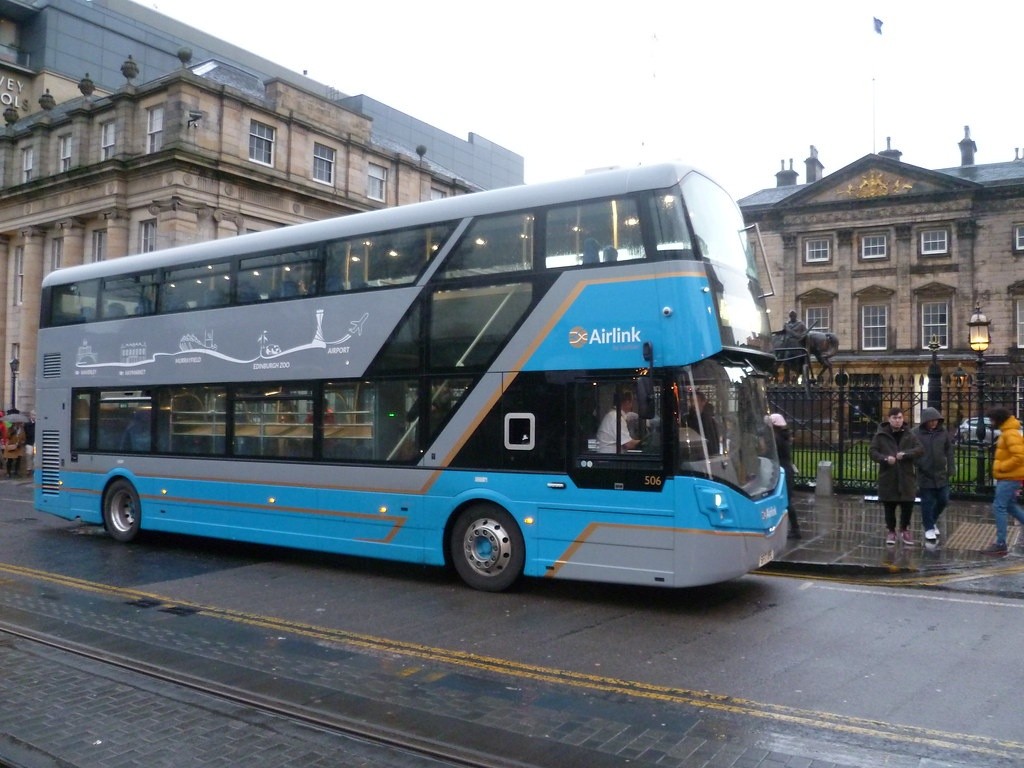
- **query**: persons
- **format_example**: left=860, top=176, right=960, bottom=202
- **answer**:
left=595, top=391, right=638, bottom=453
left=912, top=407, right=956, bottom=541
left=682, top=391, right=720, bottom=457
left=869, top=407, right=925, bottom=545
left=770, top=310, right=810, bottom=388
left=77, top=399, right=90, bottom=448
left=981, top=407, right=1024, bottom=555
left=304, top=398, right=335, bottom=424
left=0, top=410, right=35, bottom=478
left=767, top=406, right=803, bottom=540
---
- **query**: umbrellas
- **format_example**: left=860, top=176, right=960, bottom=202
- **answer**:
left=0, top=413, right=30, bottom=423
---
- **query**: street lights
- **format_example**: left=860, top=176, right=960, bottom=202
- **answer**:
left=6, top=355, right=21, bottom=415
left=964, top=298, right=994, bottom=497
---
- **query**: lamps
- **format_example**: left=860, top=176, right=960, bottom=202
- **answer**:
left=967, top=300, right=993, bottom=353
left=188, top=111, right=202, bottom=128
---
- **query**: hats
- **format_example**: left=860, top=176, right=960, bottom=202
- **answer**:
left=764, top=414, right=787, bottom=427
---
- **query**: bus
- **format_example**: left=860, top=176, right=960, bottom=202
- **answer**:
left=32, top=162, right=818, bottom=596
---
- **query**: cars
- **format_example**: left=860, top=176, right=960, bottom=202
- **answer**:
left=952, top=415, right=1024, bottom=448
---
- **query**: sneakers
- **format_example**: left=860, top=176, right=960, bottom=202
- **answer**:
left=981, top=543, right=1008, bottom=555
left=886, top=531, right=896, bottom=544
left=887, top=545, right=895, bottom=553
left=901, top=530, right=913, bottom=544
left=904, top=546, right=913, bottom=556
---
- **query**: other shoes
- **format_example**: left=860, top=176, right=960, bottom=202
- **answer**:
left=925, top=529, right=936, bottom=539
left=934, top=524, right=940, bottom=535
left=787, top=529, right=801, bottom=539
left=925, top=540, right=938, bottom=550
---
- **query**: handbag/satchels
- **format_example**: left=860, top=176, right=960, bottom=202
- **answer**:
left=3, top=445, right=18, bottom=450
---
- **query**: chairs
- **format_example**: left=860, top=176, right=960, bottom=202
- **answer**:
left=280, top=281, right=301, bottom=298
left=164, top=298, right=190, bottom=312
left=108, top=303, right=126, bottom=318
left=351, top=281, right=368, bottom=289
left=267, top=290, right=280, bottom=299
left=582, top=237, right=602, bottom=266
left=237, top=282, right=261, bottom=304
left=602, top=247, right=620, bottom=263
left=325, top=277, right=345, bottom=291
left=202, top=289, right=228, bottom=307
left=134, top=296, right=156, bottom=315
left=80, top=307, right=96, bottom=321
